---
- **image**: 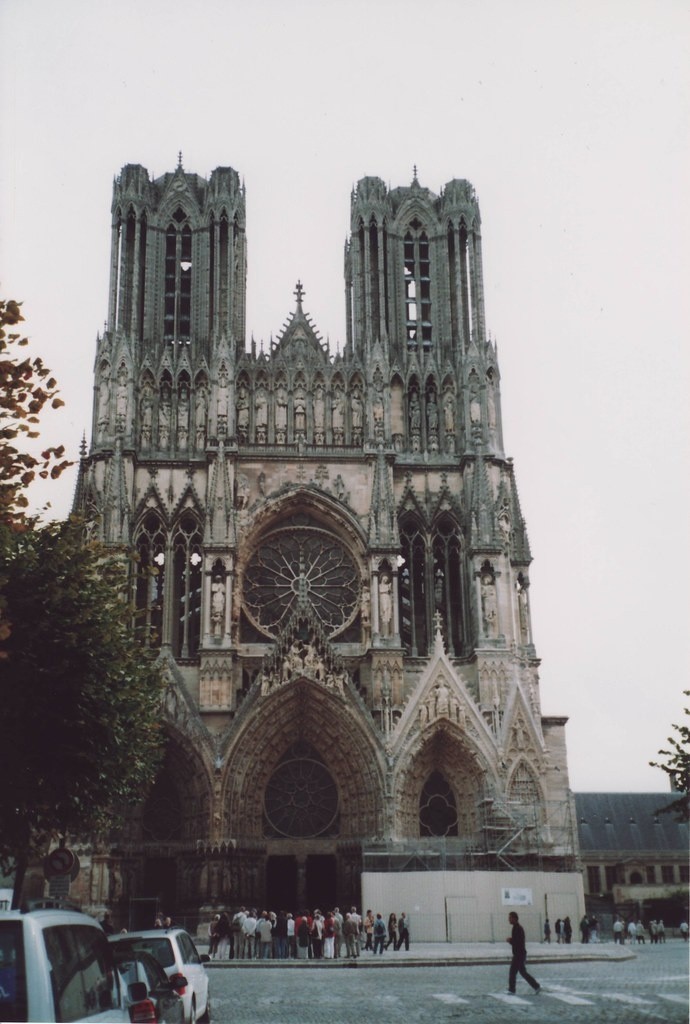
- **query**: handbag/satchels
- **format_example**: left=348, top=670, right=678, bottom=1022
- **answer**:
left=327, top=926, right=335, bottom=934
left=311, top=925, right=318, bottom=937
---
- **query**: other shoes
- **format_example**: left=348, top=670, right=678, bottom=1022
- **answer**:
left=505, top=991, right=515, bottom=995
left=535, top=986, right=543, bottom=995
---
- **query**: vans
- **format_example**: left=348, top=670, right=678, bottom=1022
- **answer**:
left=0, top=908, right=158, bottom=1023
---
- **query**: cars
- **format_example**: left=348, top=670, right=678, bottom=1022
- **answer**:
left=113, top=952, right=188, bottom=1024
left=106, top=928, right=211, bottom=1024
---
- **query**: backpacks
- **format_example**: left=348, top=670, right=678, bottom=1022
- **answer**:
left=232, top=915, right=243, bottom=931
left=376, top=924, right=383, bottom=935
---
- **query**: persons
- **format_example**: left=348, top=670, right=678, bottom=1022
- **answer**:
left=208, top=906, right=363, bottom=959
left=383, top=913, right=398, bottom=951
left=363, top=910, right=374, bottom=951
left=648, top=920, right=666, bottom=944
left=680, top=920, right=689, bottom=941
left=555, top=916, right=572, bottom=944
left=154, top=917, right=171, bottom=929
left=579, top=915, right=601, bottom=944
left=502, top=911, right=543, bottom=996
left=373, top=914, right=387, bottom=954
left=613, top=917, right=645, bottom=945
left=100, top=911, right=116, bottom=935
left=396, top=912, right=410, bottom=951
left=121, top=928, right=127, bottom=934
left=544, top=919, right=551, bottom=944
